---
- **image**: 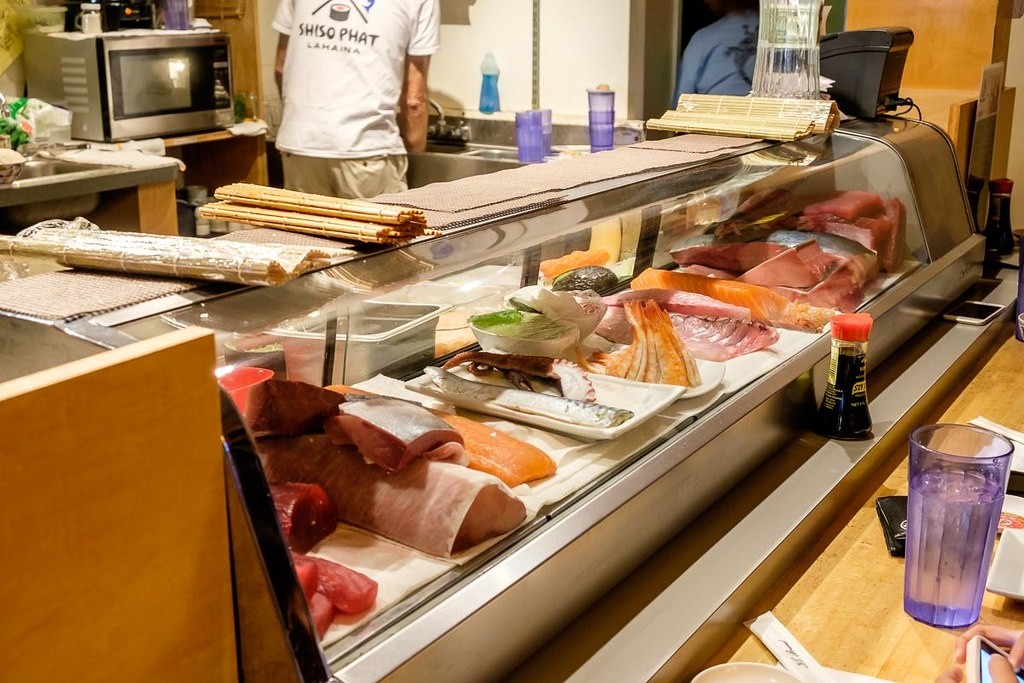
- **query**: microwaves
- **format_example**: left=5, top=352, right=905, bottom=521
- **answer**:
left=25, top=28, right=235, bottom=143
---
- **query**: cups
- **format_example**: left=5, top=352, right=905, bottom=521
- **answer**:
left=1015, top=240, right=1024, bottom=340
left=588, top=92, right=615, bottom=153
left=515, top=111, right=542, bottom=162
left=752, top=0, right=823, bottom=100
left=185, top=185, right=207, bottom=205
left=904, top=423, right=1015, bottom=628
left=163, top=0, right=189, bottom=30
left=528, top=109, right=552, bottom=156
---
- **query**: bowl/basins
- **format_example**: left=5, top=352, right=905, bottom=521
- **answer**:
left=217, top=367, right=275, bottom=428
left=1018, top=313, right=1024, bottom=341
left=0, top=148, right=26, bottom=183
left=469, top=310, right=578, bottom=358
left=503, top=285, right=607, bottom=341
left=17, top=7, right=68, bottom=33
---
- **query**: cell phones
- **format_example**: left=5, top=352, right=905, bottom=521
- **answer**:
left=876, top=495, right=930, bottom=555
left=942, top=299, right=1006, bottom=325
left=966, top=636, right=1024, bottom=683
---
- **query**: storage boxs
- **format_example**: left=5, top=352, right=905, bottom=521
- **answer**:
left=261, top=299, right=460, bottom=388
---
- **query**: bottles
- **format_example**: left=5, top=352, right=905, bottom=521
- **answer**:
left=479, top=52, right=500, bottom=111
left=81, top=3, right=101, bottom=34
left=981, top=177, right=1015, bottom=255
left=812, top=313, right=875, bottom=441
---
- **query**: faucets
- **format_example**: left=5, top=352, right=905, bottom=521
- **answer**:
left=427, top=96, right=465, bottom=139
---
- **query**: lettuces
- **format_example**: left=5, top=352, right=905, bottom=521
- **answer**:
left=466, top=308, right=523, bottom=327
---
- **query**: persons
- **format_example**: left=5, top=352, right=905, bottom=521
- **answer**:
left=671, top=1, right=762, bottom=113
left=271, top=0, right=442, bottom=198
left=934, top=624, right=1024, bottom=683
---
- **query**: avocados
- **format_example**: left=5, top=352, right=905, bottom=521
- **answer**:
left=553, top=266, right=618, bottom=293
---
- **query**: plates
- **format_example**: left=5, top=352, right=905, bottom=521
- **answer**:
left=582, top=343, right=727, bottom=399
left=997, top=494, right=1024, bottom=533
left=405, top=346, right=686, bottom=440
left=986, top=527, right=1024, bottom=600
left=691, top=662, right=803, bottom=683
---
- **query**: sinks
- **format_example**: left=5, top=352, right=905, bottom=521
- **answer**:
left=464, top=148, right=561, bottom=162
left=402, top=139, right=477, bottom=158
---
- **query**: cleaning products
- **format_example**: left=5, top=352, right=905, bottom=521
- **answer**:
left=479, top=52, right=502, bottom=115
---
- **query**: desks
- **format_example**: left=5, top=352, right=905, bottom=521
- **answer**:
left=163, top=123, right=271, bottom=236
